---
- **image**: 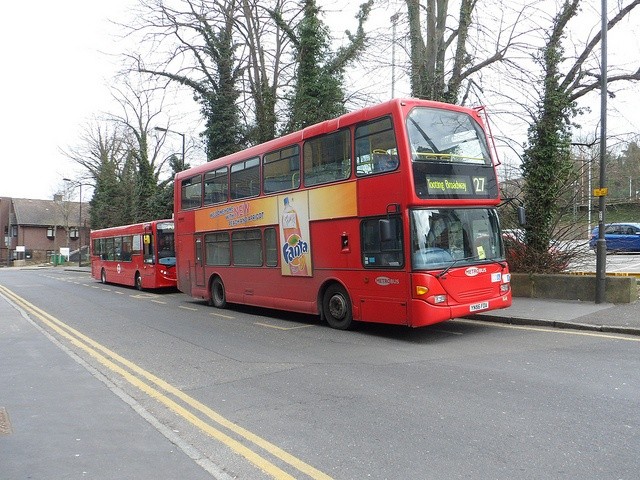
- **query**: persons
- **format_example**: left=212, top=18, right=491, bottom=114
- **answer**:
left=626, top=228, right=636, bottom=235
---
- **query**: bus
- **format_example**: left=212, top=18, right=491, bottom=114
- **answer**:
left=90, top=219, right=177, bottom=291
left=173, top=99, right=514, bottom=329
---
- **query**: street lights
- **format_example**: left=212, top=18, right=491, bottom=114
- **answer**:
left=62, top=178, right=83, bottom=267
left=156, top=126, right=186, bottom=169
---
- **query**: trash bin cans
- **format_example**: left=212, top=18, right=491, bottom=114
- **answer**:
left=59, top=255, right=65, bottom=262
left=51, top=254, right=59, bottom=262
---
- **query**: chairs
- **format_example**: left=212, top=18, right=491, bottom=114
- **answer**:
left=186, top=177, right=247, bottom=206
left=373, top=148, right=453, bottom=172
left=248, top=177, right=260, bottom=196
left=257, top=244, right=276, bottom=268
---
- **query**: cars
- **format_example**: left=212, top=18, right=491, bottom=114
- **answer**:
left=589, top=222, right=640, bottom=253
left=502, top=233, right=567, bottom=272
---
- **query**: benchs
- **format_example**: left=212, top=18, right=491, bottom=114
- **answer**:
left=264, top=174, right=292, bottom=192
left=293, top=171, right=336, bottom=189
